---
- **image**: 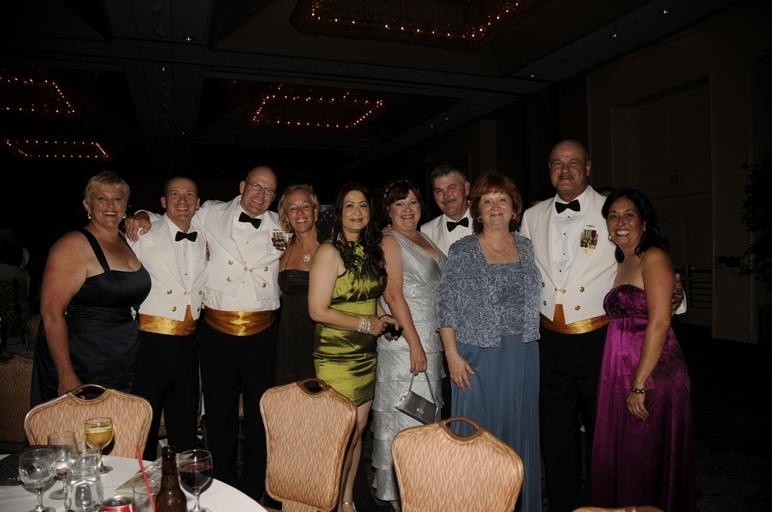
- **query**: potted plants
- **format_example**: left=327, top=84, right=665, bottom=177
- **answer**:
left=716, top=150, right=772, bottom=347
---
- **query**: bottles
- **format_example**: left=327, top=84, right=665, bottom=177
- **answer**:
left=155, top=445, right=187, bottom=512
left=101, top=497, right=137, bottom=512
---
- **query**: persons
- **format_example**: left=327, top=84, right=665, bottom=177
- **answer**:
left=123, top=168, right=294, bottom=511
left=437, top=172, right=543, bottom=511
left=591, top=185, right=690, bottom=511
left=277, top=184, right=321, bottom=394
left=420, top=167, right=474, bottom=258
left=30, top=172, right=152, bottom=406
left=519, top=139, right=686, bottom=512
left=124, top=175, right=208, bottom=460
left=307, top=187, right=399, bottom=511
left=372, top=181, right=448, bottom=511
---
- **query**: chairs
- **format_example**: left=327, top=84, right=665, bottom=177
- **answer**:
left=23, top=384, right=153, bottom=460
left=260, top=378, right=358, bottom=512
left=391, top=417, right=525, bottom=512
left=0, top=350, right=33, bottom=452
left=573, top=506, right=664, bottom=512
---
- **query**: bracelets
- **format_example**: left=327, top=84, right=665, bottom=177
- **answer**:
left=631, top=388, right=647, bottom=395
left=356, top=314, right=370, bottom=334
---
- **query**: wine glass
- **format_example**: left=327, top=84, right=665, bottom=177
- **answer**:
left=134, top=481, right=163, bottom=512
left=67, top=446, right=102, bottom=473
left=48, top=432, right=79, bottom=501
left=180, top=451, right=212, bottom=512
left=84, top=418, right=113, bottom=473
left=62, top=466, right=104, bottom=512
left=19, top=450, right=56, bottom=512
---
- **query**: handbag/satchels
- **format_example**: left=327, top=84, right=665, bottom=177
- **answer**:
left=399, top=369, right=436, bottom=426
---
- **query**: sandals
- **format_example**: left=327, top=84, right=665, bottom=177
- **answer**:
left=344, top=501, right=357, bottom=510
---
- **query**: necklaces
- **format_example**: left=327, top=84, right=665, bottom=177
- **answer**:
left=295, top=243, right=319, bottom=264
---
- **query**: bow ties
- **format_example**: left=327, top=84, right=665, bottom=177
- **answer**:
left=554, top=200, right=581, bottom=213
left=447, top=217, right=469, bottom=231
left=238, top=212, right=262, bottom=227
left=175, top=231, right=198, bottom=242
